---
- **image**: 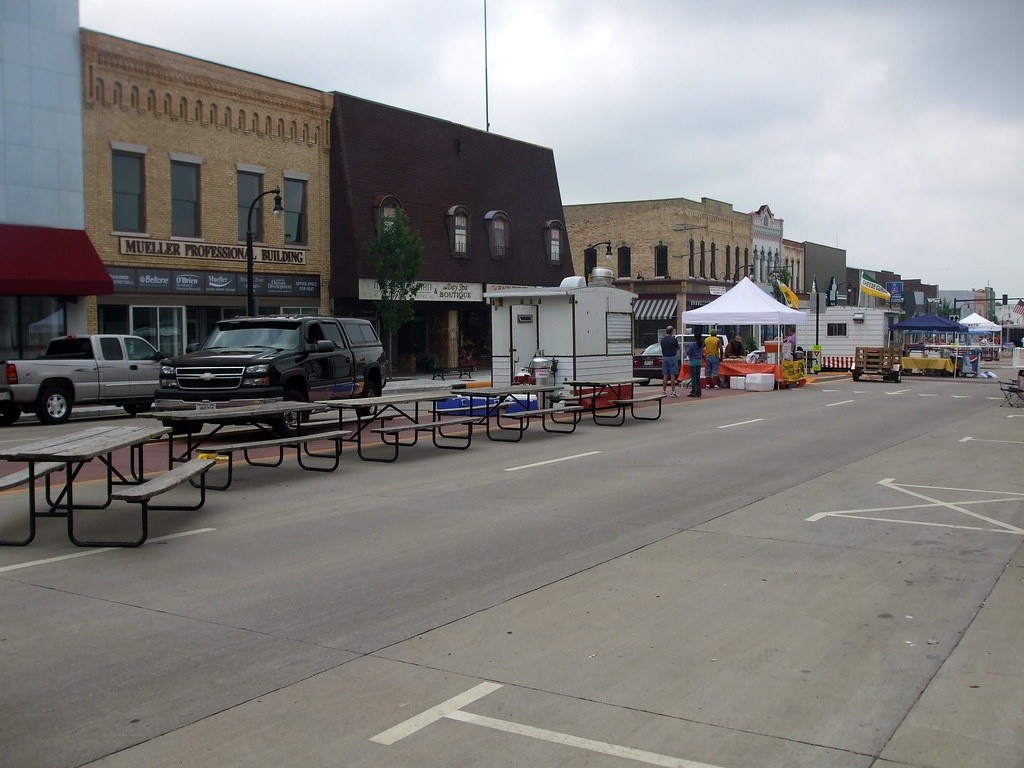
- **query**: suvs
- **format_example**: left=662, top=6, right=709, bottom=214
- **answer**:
left=154, top=314, right=388, bottom=436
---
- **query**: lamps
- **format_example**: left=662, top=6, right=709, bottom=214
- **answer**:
left=637, top=274, right=644, bottom=280
left=699, top=269, right=744, bottom=282
left=665, top=275, right=671, bottom=279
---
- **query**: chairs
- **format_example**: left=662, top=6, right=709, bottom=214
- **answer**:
left=998, top=378, right=1024, bottom=409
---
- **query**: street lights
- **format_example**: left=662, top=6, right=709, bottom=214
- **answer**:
left=958, top=303, right=970, bottom=321
left=246, top=186, right=284, bottom=314
left=986, top=309, right=995, bottom=319
left=890, top=291, right=905, bottom=309
left=1001, top=312, right=1011, bottom=325
left=733, top=264, right=756, bottom=339
left=929, top=298, right=942, bottom=313
left=835, top=280, right=852, bottom=306
left=588, top=240, right=614, bottom=276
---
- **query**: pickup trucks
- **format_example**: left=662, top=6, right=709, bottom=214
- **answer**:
left=0, top=335, right=168, bottom=425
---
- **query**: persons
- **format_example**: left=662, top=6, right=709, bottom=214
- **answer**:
left=774, top=327, right=795, bottom=353
left=660, top=326, right=681, bottom=398
left=462, top=338, right=475, bottom=362
left=994, top=334, right=1000, bottom=345
left=704, top=329, right=722, bottom=390
left=725, top=336, right=745, bottom=358
left=686, top=331, right=703, bottom=397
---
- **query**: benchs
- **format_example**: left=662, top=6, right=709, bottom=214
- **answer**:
left=432, top=358, right=476, bottom=381
left=0, top=388, right=668, bottom=548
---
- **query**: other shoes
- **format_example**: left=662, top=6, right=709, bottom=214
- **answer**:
left=714, top=385, right=720, bottom=390
left=706, top=384, right=710, bottom=390
left=687, top=393, right=697, bottom=397
left=663, top=391, right=667, bottom=397
left=670, top=391, right=679, bottom=398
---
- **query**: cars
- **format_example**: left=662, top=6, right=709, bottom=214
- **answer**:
left=633, top=342, right=692, bottom=386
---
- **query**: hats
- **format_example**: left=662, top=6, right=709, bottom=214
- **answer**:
left=735, top=336, right=742, bottom=343
left=710, top=329, right=717, bottom=334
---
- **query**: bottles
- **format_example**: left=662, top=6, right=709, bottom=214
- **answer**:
left=892, top=284, right=898, bottom=293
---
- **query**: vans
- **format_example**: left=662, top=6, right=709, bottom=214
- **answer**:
left=674, top=334, right=728, bottom=359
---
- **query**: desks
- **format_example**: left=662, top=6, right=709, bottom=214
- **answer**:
left=314, top=394, right=458, bottom=457
left=677, top=362, right=805, bottom=388
left=451, top=385, right=563, bottom=440
left=136, top=402, right=326, bottom=487
left=0, top=426, right=172, bottom=548
left=901, top=356, right=954, bottom=378
left=562, top=377, right=650, bottom=423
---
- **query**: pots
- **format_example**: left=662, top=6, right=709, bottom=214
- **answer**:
left=793, top=350, right=804, bottom=359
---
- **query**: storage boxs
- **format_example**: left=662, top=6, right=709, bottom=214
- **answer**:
left=505, top=394, right=537, bottom=413
left=701, top=368, right=715, bottom=388
left=437, top=395, right=466, bottom=415
left=462, top=396, right=499, bottom=417
left=730, top=374, right=776, bottom=391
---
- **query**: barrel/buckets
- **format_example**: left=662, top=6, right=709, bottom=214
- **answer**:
left=700, top=367, right=706, bottom=378
left=553, top=400, right=565, bottom=413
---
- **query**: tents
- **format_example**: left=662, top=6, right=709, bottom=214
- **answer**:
left=887, top=314, right=968, bottom=379
left=682, top=276, right=807, bottom=393
left=946, top=312, right=1002, bottom=362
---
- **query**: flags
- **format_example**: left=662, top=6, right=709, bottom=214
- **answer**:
left=1013, top=298, right=1024, bottom=315
left=862, top=272, right=890, bottom=300
left=777, top=279, right=800, bottom=308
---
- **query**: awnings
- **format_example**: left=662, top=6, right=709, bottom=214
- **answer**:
left=633, top=299, right=678, bottom=320
left=0, top=222, right=114, bottom=295
left=689, top=300, right=710, bottom=305
